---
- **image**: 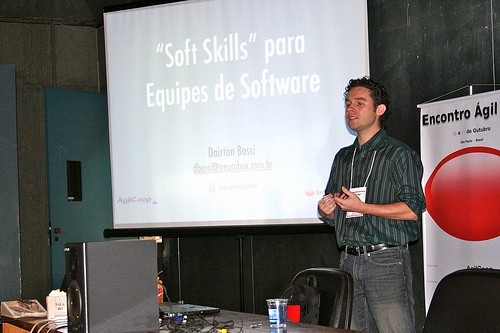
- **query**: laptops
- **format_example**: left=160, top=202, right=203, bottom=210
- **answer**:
left=161, top=304, right=220, bottom=316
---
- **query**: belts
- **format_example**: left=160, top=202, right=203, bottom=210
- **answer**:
left=341, top=242, right=389, bottom=255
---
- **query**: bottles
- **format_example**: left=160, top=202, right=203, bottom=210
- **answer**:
left=158, top=276, right=164, bottom=303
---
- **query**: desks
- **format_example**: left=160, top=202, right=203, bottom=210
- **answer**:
left=0, top=302, right=371, bottom=333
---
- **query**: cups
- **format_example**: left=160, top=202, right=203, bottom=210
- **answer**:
left=266, top=298, right=289, bottom=330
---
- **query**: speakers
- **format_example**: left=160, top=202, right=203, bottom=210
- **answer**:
left=65, top=239, right=159, bottom=333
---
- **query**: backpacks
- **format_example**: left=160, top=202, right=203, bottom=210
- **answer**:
left=276, top=274, right=320, bottom=326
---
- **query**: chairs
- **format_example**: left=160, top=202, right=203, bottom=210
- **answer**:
left=423, top=268, right=500, bottom=333
left=289, top=268, right=355, bottom=330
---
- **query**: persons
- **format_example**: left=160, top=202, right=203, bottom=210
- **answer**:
left=317, top=77, right=426, bottom=333
